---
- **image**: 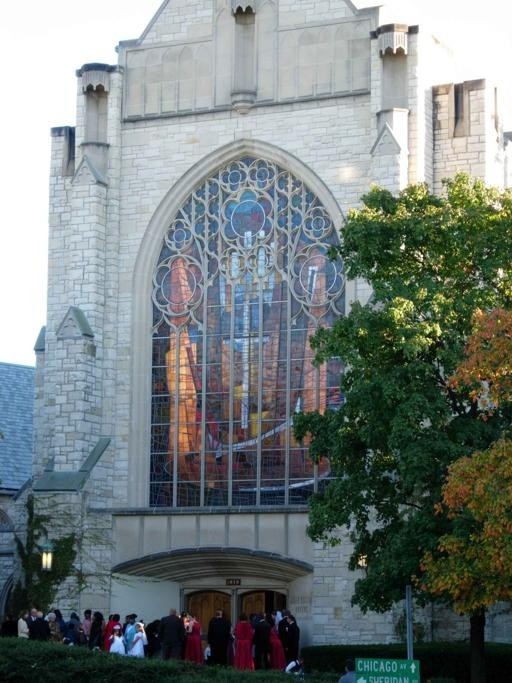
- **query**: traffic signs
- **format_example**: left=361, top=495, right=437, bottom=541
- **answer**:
left=355, top=674, right=420, bottom=683
left=355, top=659, right=420, bottom=676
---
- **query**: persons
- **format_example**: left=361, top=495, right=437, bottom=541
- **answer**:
left=145, top=608, right=300, bottom=674
left=338, top=659, right=356, bottom=682
left=2, top=607, right=148, bottom=657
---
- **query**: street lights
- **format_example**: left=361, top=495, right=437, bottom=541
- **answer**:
left=38, top=543, right=57, bottom=614
